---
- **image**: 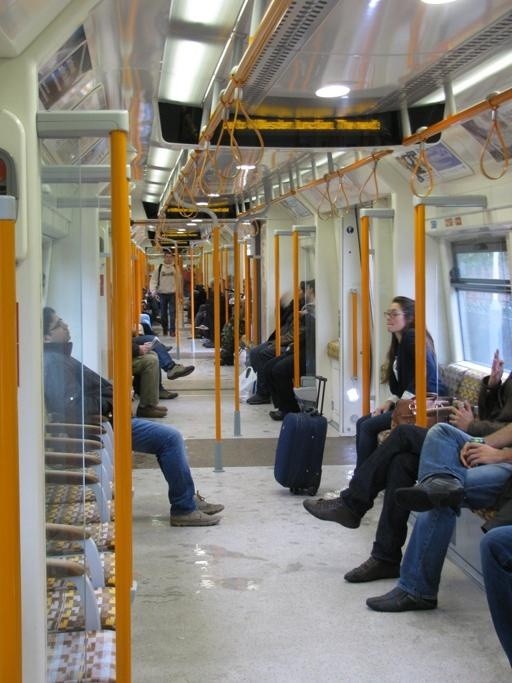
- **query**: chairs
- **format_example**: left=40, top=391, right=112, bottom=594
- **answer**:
left=32, top=403, right=118, bottom=683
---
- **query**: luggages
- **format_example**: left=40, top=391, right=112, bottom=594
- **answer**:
left=274, top=374, right=328, bottom=495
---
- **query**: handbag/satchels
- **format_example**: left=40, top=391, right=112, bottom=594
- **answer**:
left=391, top=392, right=454, bottom=436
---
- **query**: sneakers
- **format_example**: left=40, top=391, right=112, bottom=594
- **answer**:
left=167, top=364, right=194, bottom=379
left=159, top=383, right=177, bottom=398
left=136, top=406, right=166, bottom=416
left=155, top=405, right=168, bottom=411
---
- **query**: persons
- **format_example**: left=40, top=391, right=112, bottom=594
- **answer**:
left=149, top=252, right=183, bottom=337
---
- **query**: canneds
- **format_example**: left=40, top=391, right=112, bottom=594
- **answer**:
left=470, top=437, right=486, bottom=467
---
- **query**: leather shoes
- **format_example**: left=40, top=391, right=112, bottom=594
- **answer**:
left=393, top=477, right=471, bottom=512
left=365, top=588, right=437, bottom=612
left=170, top=506, right=223, bottom=527
left=304, top=497, right=361, bottom=529
left=344, top=557, right=400, bottom=583
left=270, top=410, right=283, bottom=420
left=246, top=394, right=271, bottom=405
left=191, top=490, right=224, bottom=514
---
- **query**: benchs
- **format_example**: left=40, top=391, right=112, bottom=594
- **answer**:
left=377, top=357, right=509, bottom=586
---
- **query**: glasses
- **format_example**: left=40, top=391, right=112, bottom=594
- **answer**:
left=383, top=311, right=401, bottom=320
left=49, top=318, right=64, bottom=333
